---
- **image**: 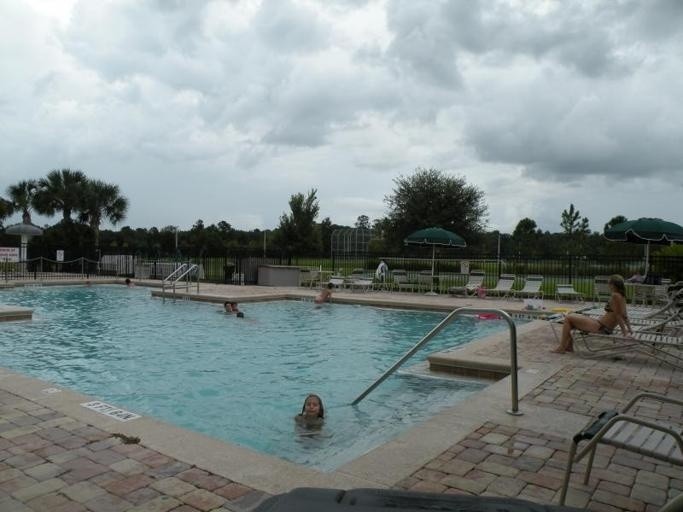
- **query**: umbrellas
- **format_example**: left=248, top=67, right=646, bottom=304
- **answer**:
left=602, top=217, right=682, bottom=281
left=400, top=226, right=466, bottom=293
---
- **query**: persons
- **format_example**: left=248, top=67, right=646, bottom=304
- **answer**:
left=626, top=271, right=642, bottom=283
left=124, top=278, right=135, bottom=289
left=236, top=312, right=244, bottom=322
left=373, top=260, right=388, bottom=291
left=314, top=282, right=334, bottom=304
left=222, top=301, right=237, bottom=315
left=547, top=273, right=633, bottom=355
left=292, top=393, right=324, bottom=433
left=230, top=302, right=241, bottom=313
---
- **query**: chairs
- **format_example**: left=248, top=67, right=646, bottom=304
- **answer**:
left=306, top=268, right=430, bottom=295
left=548, top=275, right=683, bottom=373
left=448, top=274, right=545, bottom=302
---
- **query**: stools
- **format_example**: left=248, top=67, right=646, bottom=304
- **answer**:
left=561, top=392, right=683, bottom=511
